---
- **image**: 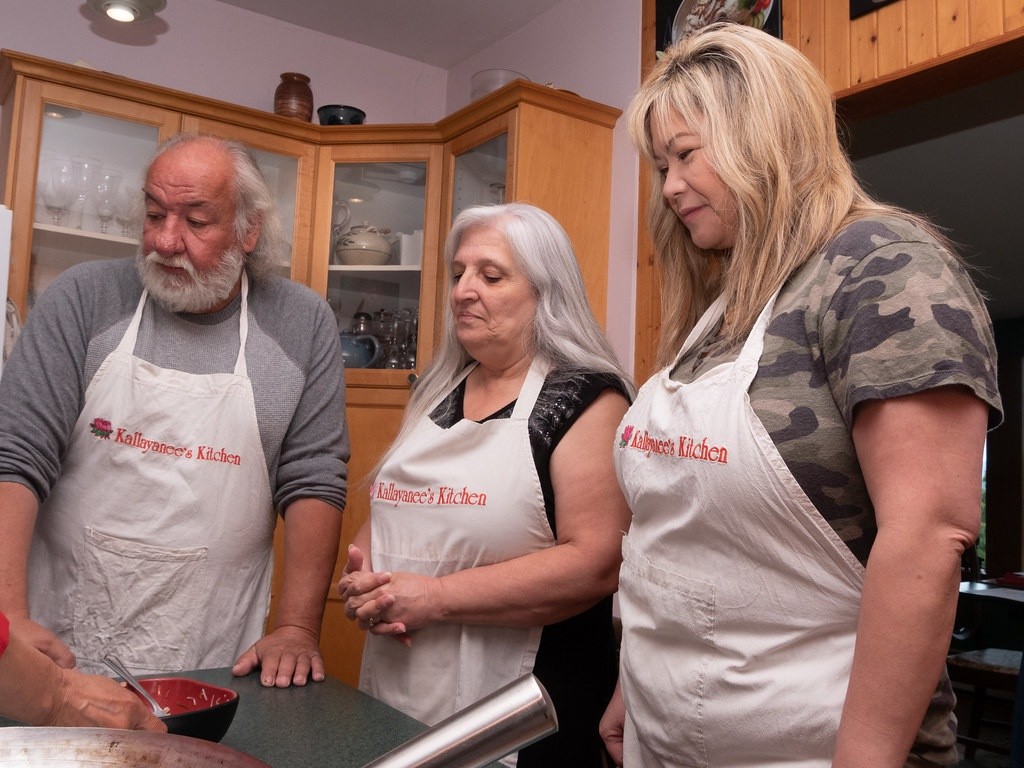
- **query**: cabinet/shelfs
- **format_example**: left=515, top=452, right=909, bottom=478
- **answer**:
left=0, top=1, right=1024, bottom=685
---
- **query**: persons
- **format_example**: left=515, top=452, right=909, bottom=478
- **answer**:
left=600, top=22, right=1005, bottom=768
left=0, top=137, right=352, bottom=688
left=337, top=201, right=638, bottom=768
left=0, top=612, right=168, bottom=733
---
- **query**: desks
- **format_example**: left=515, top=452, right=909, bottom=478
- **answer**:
left=0, top=668, right=518, bottom=768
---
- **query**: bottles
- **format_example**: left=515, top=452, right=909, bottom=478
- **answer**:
left=274, top=70, right=313, bottom=123
left=350, top=305, right=417, bottom=369
left=335, top=220, right=392, bottom=264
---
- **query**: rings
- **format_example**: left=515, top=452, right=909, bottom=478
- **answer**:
left=369, top=618, right=376, bottom=627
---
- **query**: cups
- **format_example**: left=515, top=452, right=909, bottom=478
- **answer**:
left=329, top=198, right=351, bottom=263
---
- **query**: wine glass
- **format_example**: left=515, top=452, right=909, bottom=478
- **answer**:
left=35, top=154, right=146, bottom=240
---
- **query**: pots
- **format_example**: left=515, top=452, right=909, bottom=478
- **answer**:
left=0, top=674, right=558, bottom=768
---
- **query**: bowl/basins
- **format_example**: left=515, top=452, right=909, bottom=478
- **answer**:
left=118, top=677, right=239, bottom=744
left=469, top=67, right=530, bottom=102
left=316, top=104, right=367, bottom=125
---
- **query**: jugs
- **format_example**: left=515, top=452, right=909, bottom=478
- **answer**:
left=338, top=334, right=380, bottom=369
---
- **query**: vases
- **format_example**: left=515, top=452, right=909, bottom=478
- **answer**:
left=274, top=72, right=312, bottom=122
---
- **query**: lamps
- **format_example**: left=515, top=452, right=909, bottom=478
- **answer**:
left=86, top=0, right=167, bottom=24
